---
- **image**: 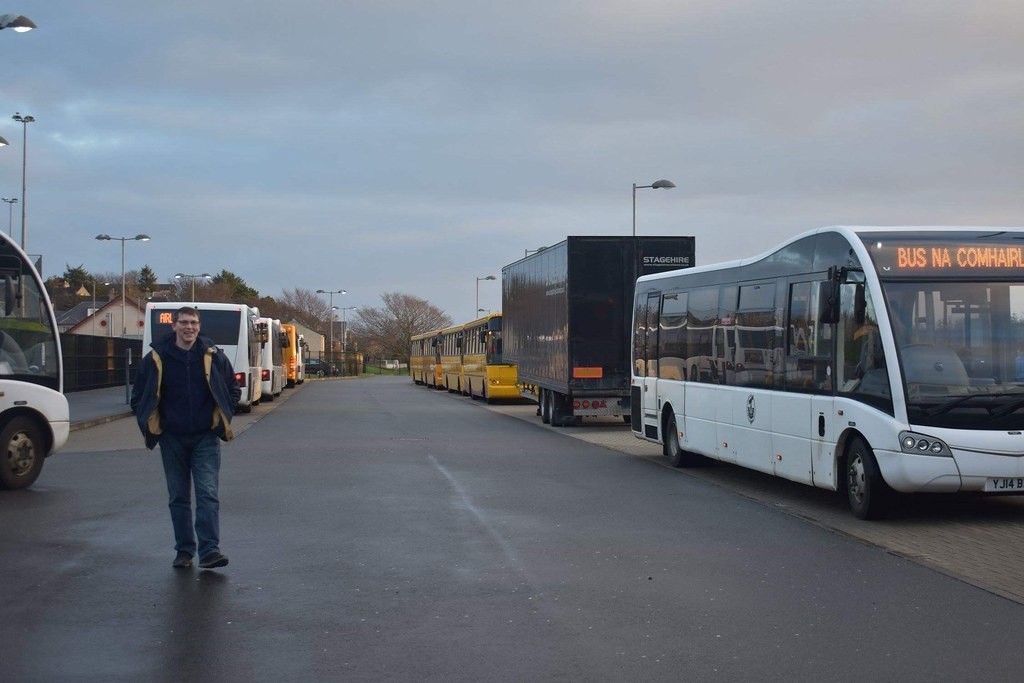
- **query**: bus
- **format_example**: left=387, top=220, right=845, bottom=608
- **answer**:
left=254, top=318, right=291, bottom=402
left=0, top=231, right=72, bottom=493
left=296, top=334, right=309, bottom=383
left=280, top=324, right=305, bottom=388
left=375, top=359, right=399, bottom=370
left=629, top=228, right=1024, bottom=520
left=409, top=314, right=540, bottom=404
left=141, top=301, right=269, bottom=415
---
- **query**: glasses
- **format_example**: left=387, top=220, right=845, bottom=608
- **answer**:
left=176, top=320, right=199, bottom=326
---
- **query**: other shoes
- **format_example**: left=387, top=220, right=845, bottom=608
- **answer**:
left=198, top=552, right=229, bottom=568
left=173, top=552, right=194, bottom=568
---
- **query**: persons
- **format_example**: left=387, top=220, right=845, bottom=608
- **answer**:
left=130, top=307, right=242, bottom=568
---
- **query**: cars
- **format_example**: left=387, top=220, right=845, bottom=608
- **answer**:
left=304, top=359, right=340, bottom=377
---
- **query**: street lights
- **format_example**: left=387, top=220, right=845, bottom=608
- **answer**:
left=477, top=308, right=491, bottom=314
left=332, top=305, right=357, bottom=352
left=475, top=274, right=496, bottom=320
left=525, top=246, right=549, bottom=258
left=96, top=234, right=151, bottom=340
left=316, top=289, right=348, bottom=377
left=2, top=197, right=20, bottom=238
left=174, top=273, right=212, bottom=303
left=13, top=111, right=36, bottom=252
left=633, top=180, right=676, bottom=236
